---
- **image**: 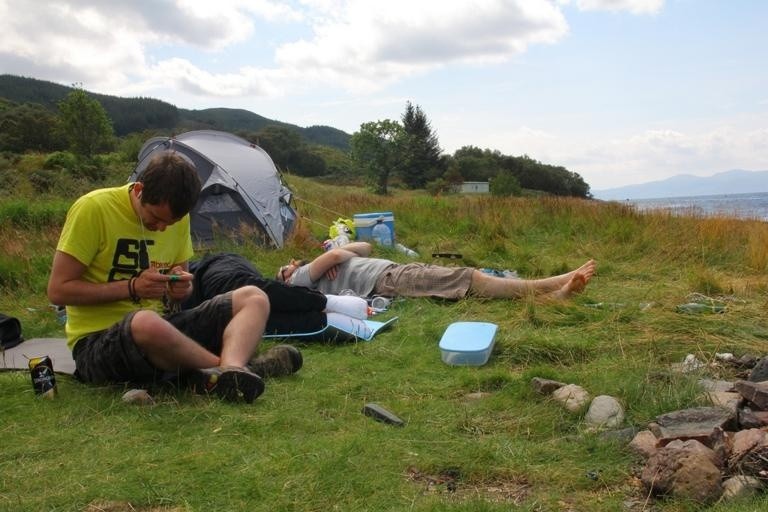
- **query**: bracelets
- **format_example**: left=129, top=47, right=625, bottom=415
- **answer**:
left=128, top=276, right=140, bottom=305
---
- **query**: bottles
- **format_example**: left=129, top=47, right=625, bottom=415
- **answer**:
left=394, top=243, right=420, bottom=261
left=373, top=218, right=393, bottom=251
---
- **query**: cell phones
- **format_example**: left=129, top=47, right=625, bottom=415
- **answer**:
left=160, top=274, right=179, bottom=280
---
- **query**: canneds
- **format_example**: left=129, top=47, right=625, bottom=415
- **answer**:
left=29, top=355, right=58, bottom=400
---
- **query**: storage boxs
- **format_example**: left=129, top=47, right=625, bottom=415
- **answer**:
left=352, top=212, right=397, bottom=249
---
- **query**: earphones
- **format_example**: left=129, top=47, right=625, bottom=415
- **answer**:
left=137, top=191, right=143, bottom=197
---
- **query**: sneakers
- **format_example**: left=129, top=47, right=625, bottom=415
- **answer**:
left=191, top=364, right=266, bottom=403
left=247, top=344, right=302, bottom=375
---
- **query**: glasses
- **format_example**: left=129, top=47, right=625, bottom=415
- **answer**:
left=281, top=264, right=288, bottom=281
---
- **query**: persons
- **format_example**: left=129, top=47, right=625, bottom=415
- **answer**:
left=277, top=241, right=596, bottom=303
left=158, top=252, right=371, bottom=344
left=48, top=152, right=303, bottom=402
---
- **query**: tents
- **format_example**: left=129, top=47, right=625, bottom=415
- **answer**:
left=129, top=130, right=298, bottom=250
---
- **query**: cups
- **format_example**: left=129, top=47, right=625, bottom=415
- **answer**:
left=372, top=297, right=390, bottom=311
